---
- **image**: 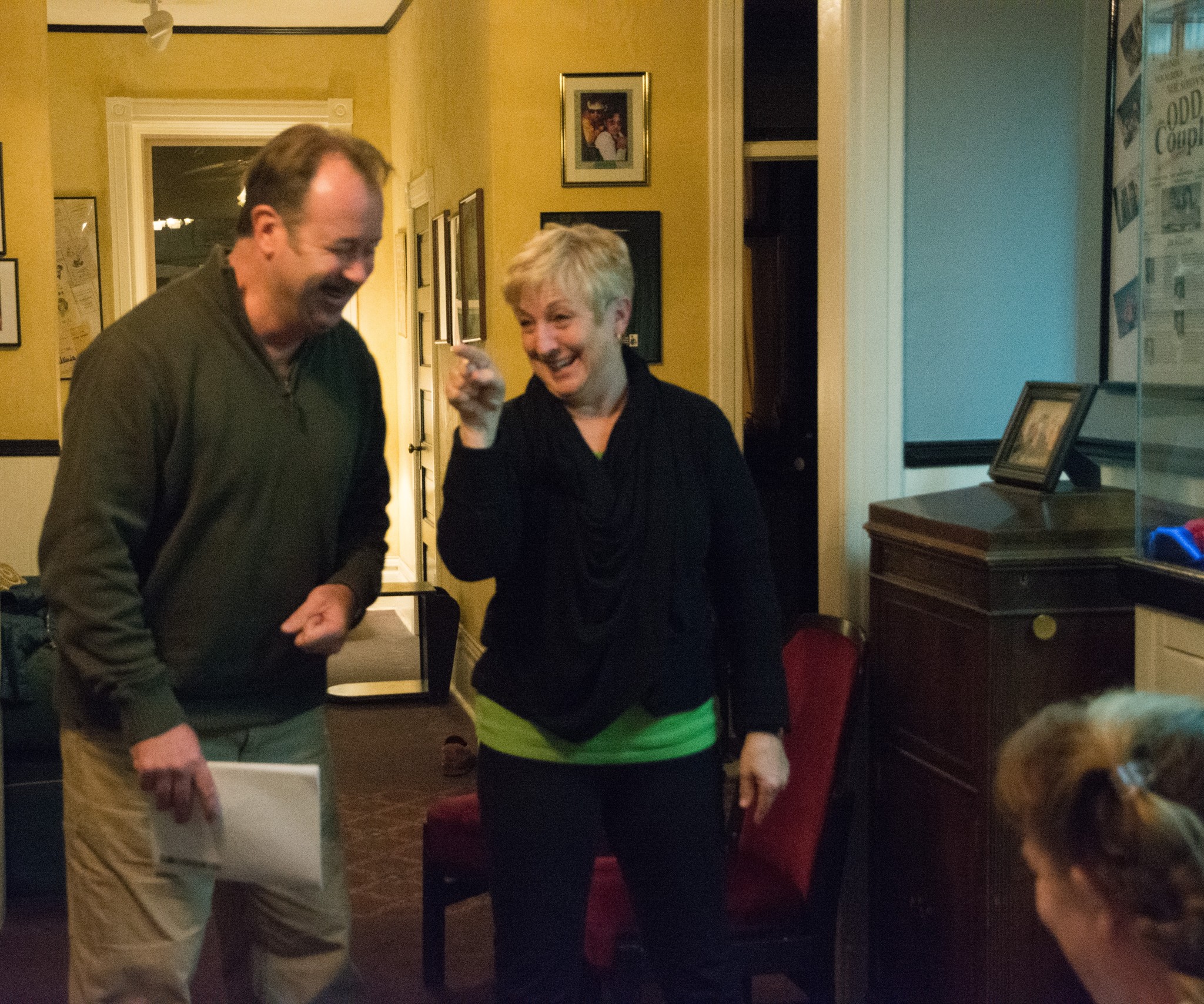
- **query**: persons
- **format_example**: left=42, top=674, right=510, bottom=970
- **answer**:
left=36, top=118, right=390, bottom=1004
left=1107, top=0, right=1203, bottom=381
left=592, top=105, right=629, bottom=160
left=435, top=222, right=794, bottom=1004
left=581, top=95, right=616, bottom=158
left=986, top=688, right=1204, bottom=1004
left=1017, top=413, right=1052, bottom=464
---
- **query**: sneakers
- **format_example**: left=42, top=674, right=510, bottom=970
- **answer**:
left=441, top=734, right=476, bottom=776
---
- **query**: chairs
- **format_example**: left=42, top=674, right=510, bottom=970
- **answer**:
left=580, top=613, right=873, bottom=998
left=418, top=796, right=499, bottom=1004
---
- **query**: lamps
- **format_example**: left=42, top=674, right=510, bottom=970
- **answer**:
left=132, top=0, right=175, bottom=55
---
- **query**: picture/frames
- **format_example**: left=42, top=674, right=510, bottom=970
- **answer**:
left=458, top=185, right=487, bottom=345
left=429, top=208, right=452, bottom=346
left=1, top=256, right=21, bottom=349
left=1096, top=1, right=1201, bottom=401
left=444, top=210, right=465, bottom=351
left=54, top=194, right=103, bottom=382
left=560, top=70, right=652, bottom=189
left=987, top=379, right=1102, bottom=491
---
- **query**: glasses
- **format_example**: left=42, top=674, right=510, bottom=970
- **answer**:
left=588, top=108, right=605, bottom=114
